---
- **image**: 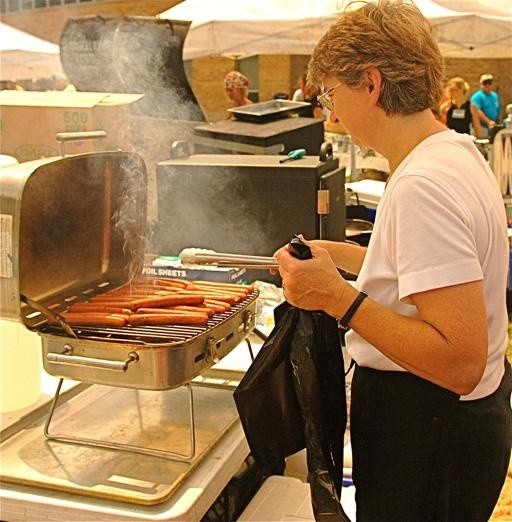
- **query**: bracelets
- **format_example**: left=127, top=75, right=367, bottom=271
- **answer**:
left=336, top=289, right=368, bottom=330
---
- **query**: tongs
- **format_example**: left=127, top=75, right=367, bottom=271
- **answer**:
left=180, top=247, right=280, bottom=271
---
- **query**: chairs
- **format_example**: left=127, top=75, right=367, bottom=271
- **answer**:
left=490, top=128, right=512, bottom=206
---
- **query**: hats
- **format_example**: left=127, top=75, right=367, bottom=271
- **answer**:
left=479, top=74, right=493, bottom=83
left=225, top=71, right=248, bottom=89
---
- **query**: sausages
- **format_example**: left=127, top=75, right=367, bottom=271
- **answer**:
left=48, top=278, right=187, bottom=327
left=128, top=281, right=255, bottom=324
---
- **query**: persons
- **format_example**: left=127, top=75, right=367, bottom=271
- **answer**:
left=469, top=74, right=502, bottom=138
left=274, top=0, right=511, bottom=522
left=440, top=77, right=482, bottom=139
left=225, top=71, right=253, bottom=121
left=291, top=73, right=331, bottom=119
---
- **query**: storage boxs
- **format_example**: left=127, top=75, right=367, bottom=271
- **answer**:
left=235, top=474, right=357, bottom=522
left=1, top=88, right=145, bottom=165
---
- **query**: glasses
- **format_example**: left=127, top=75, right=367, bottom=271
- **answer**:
left=316, top=81, right=343, bottom=113
left=483, top=81, right=492, bottom=85
